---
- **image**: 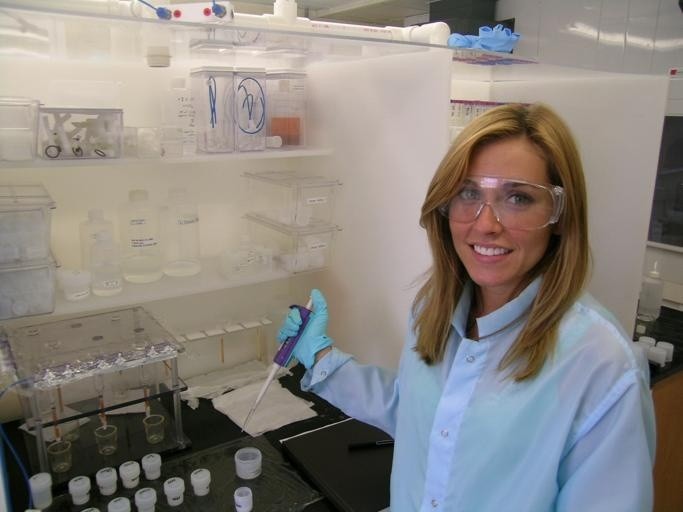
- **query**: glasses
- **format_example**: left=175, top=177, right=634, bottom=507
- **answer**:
left=437, top=174, right=565, bottom=230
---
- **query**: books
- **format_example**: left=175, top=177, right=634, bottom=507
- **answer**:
left=280, top=418, right=395, bottom=512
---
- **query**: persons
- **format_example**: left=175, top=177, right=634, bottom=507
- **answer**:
left=274, top=101, right=660, bottom=512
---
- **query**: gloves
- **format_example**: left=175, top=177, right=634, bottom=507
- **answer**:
left=277, top=289, right=333, bottom=369
left=447, top=23, right=520, bottom=52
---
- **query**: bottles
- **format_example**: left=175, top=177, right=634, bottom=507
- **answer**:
left=28, top=447, right=262, bottom=512
left=164, top=76, right=199, bottom=157
left=637, top=263, right=664, bottom=321
left=58, top=182, right=204, bottom=300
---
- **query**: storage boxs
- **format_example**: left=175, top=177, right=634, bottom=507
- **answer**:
left=1, top=183, right=62, bottom=319
left=1, top=97, right=123, bottom=157
left=241, top=169, right=342, bottom=273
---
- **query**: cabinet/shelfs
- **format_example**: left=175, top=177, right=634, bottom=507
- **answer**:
left=1, top=148, right=335, bottom=333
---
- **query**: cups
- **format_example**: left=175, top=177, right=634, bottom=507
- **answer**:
left=142, top=414, right=165, bottom=444
left=46, top=441, right=71, bottom=474
left=94, top=425, right=117, bottom=456
left=186, top=65, right=308, bottom=154
